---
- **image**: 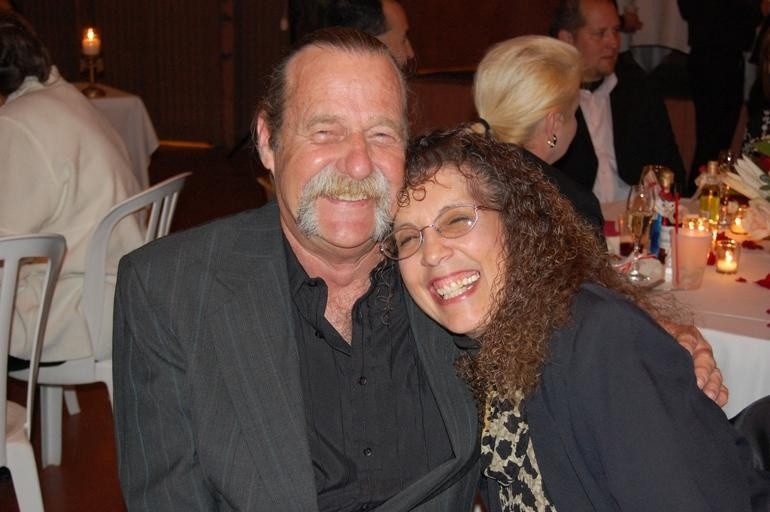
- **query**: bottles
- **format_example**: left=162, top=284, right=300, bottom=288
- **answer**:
left=699, top=150, right=732, bottom=230
left=654, top=170, right=680, bottom=265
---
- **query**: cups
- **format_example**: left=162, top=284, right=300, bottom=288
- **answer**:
left=617, top=214, right=634, bottom=260
left=668, top=227, right=713, bottom=291
left=716, top=239, right=740, bottom=275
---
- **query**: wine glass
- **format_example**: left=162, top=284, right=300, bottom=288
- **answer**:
left=626, top=184, right=654, bottom=258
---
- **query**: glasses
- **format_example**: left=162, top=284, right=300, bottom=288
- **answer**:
left=380, top=205, right=509, bottom=261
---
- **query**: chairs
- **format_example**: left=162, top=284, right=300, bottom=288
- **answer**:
left=0, top=235, right=70, bottom=510
left=7, top=170, right=201, bottom=467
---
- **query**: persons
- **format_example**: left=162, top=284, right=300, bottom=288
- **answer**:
left=463, top=34, right=604, bottom=235
left=112, top=24, right=729, bottom=512
left=281, top=0, right=408, bottom=59
left=0, top=0, right=149, bottom=375
left=382, top=128, right=770, bottom=512
left=544, top=1, right=769, bottom=191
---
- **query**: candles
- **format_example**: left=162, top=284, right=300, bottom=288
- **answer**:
left=83, top=28, right=103, bottom=55
